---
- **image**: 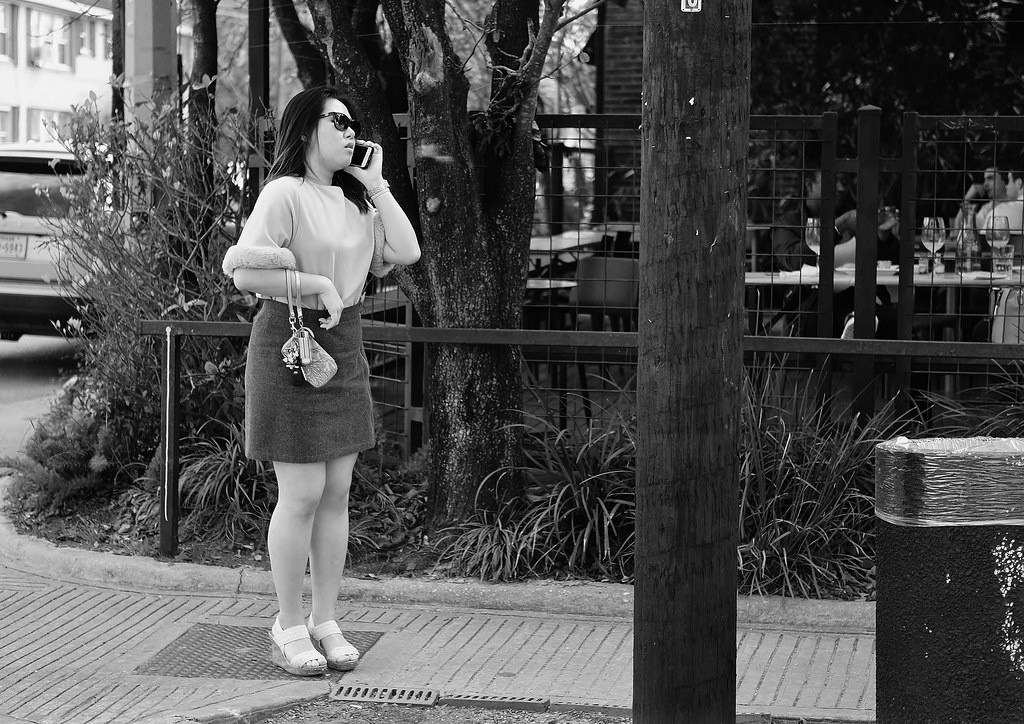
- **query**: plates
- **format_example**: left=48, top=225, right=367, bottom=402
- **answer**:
left=836, top=266, right=900, bottom=276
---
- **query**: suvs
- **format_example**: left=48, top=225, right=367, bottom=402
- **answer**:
left=0, top=140, right=181, bottom=347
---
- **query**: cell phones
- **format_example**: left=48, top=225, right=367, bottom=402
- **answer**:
left=350, top=144, right=374, bottom=169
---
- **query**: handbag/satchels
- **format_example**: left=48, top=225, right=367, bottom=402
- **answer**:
left=281, top=269, right=338, bottom=388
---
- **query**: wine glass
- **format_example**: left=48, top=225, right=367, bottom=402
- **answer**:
left=986, top=217, right=1010, bottom=273
left=806, top=218, right=820, bottom=278
left=921, top=217, right=946, bottom=276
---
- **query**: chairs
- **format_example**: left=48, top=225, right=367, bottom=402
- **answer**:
left=744, top=269, right=1024, bottom=415
left=593, top=235, right=615, bottom=257
left=569, top=255, right=639, bottom=310
left=613, top=231, right=632, bottom=258
left=978, top=234, right=1024, bottom=272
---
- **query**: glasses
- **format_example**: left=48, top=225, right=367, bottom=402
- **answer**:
left=318, top=112, right=362, bottom=139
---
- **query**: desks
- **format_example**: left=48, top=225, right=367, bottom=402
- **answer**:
left=556, top=232, right=640, bottom=245
left=529, top=237, right=592, bottom=255
left=525, top=279, right=577, bottom=441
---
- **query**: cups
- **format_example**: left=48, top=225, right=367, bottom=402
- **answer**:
left=993, top=245, right=1014, bottom=276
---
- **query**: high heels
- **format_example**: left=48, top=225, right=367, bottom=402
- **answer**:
left=308, top=612, right=360, bottom=671
left=272, top=615, right=327, bottom=675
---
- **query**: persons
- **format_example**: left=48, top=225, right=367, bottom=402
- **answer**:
left=222, top=88, right=421, bottom=674
left=769, top=158, right=1024, bottom=340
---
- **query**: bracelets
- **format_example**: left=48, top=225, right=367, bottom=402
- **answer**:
left=367, top=180, right=390, bottom=200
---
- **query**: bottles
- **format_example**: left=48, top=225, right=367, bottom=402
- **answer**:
left=955, top=204, right=982, bottom=277
